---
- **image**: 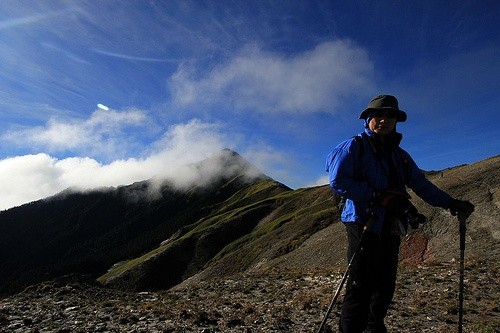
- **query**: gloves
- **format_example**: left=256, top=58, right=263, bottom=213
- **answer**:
left=450, top=200, right=474, bottom=221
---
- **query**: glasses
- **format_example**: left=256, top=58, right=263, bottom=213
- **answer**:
left=370, top=111, right=397, bottom=119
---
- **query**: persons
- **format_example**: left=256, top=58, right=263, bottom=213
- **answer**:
left=325, top=95, right=476, bottom=333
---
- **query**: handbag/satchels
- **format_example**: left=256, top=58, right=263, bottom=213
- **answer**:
left=379, top=195, right=402, bottom=211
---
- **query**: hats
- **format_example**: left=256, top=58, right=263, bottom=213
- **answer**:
left=358, top=95, right=407, bottom=122
